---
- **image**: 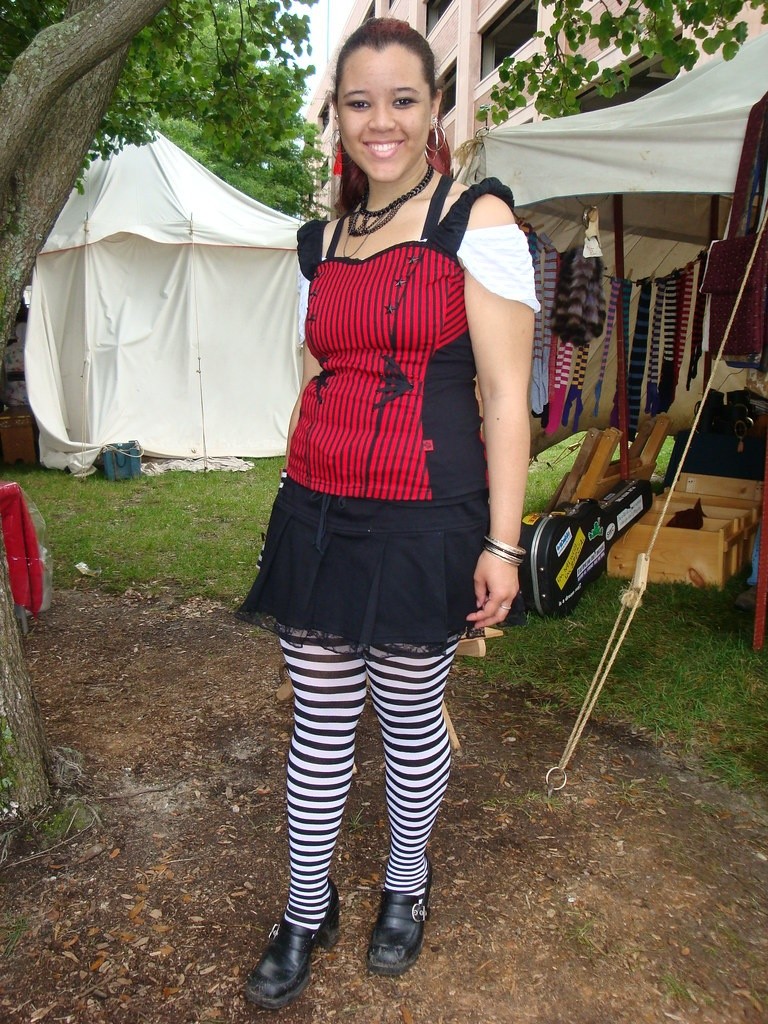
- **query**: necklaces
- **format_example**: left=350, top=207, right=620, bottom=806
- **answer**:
left=342, top=163, right=434, bottom=259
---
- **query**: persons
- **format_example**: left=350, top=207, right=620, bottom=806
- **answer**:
left=235, top=14, right=542, bottom=1010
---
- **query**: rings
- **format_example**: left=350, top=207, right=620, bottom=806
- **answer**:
left=501, top=605, right=511, bottom=610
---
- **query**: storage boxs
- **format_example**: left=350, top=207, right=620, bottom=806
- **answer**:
left=605, top=472, right=765, bottom=591
left=103, top=440, right=141, bottom=482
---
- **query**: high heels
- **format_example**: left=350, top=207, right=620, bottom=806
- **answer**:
left=366, top=851, right=432, bottom=978
left=243, top=876, right=340, bottom=1009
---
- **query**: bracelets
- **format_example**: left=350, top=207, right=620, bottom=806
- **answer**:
left=482, top=535, right=526, bottom=567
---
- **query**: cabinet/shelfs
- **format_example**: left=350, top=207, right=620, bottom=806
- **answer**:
left=0, top=405, right=37, bottom=466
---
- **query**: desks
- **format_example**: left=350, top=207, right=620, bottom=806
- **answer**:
left=661, top=431, right=766, bottom=487
left=545, top=414, right=674, bottom=512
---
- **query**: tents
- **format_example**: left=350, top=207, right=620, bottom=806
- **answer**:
left=454, top=24, right=768, bottom=470
left=24, top=120, right=312, bottom=480
